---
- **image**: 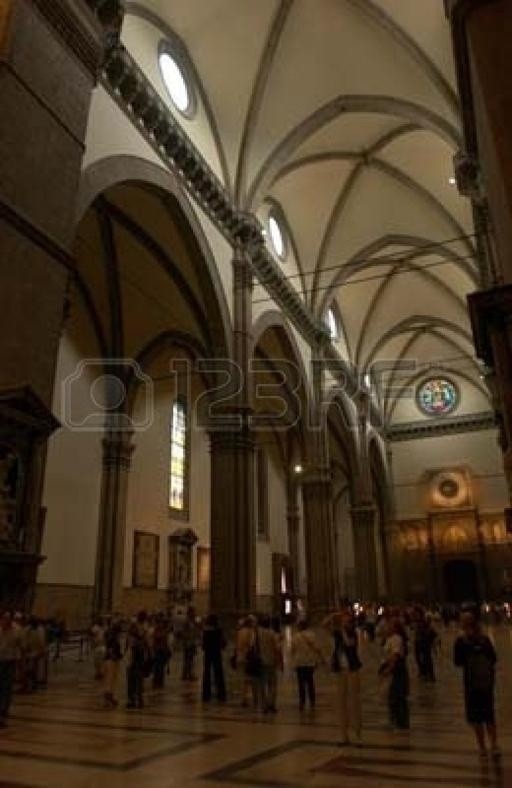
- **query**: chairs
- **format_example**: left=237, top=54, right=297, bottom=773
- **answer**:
left=52, top=611, right=93, bottom=663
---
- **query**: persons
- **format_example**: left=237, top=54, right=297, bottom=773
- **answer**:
left=2, top=597, right=511, bottom=762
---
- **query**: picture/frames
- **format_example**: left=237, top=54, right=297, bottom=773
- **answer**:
left=197, top=545, right=211, bottom=594
left=133, top=528, right=159, bottom=591
